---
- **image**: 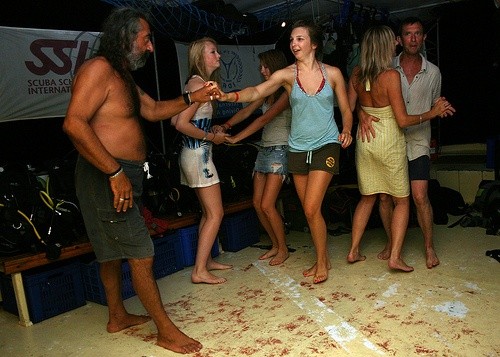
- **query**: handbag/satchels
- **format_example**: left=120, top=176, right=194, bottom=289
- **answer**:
left=0, top=160, right=87, bottom=260
left=283, top=190, right=329, bottom=233
left=141, top=167, right=182, bottom=218
left=327, top=178, right=467, bottom=230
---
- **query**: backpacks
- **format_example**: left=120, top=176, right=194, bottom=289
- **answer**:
left=447, top=179, right=500, bottom=228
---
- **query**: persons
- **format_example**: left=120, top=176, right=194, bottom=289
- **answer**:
left=62, top=8, right=222, bottom=353
left=212, top=50, right=292, bottom=265
left=355, top=16, right=456, bottom=270
left=204, top=20, right=352, bottom=283
left=171, top=37, right=232, bottom=284
left=347, top=26, right=451, bottom=271
left=323, top=36, right=350, bottom=83
left=325, top=37, right=336, bottom=53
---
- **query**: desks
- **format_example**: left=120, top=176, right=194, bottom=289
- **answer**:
left=0, top=188, right=292, bottom=327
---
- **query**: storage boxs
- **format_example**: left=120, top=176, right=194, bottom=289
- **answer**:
left=0, top=261, right=85, bottom=325
left=82, top=261, right=136, bottom=305
left=153, top=232, right=184, bottom=280
left=177, top=225, right=219, bottom=267
left=220, top=211, right=260, bottom=252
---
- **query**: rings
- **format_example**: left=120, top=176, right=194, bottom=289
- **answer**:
left=119, top=198, right=129, bottom=201
left=443, top=103, right=445, bottom=106
left=210, top=96, right=213, bottom=101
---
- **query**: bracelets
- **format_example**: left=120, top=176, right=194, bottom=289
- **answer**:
left=226, top=122, right=231, bottom=129
left=221, top=125, right=226, bottom=132
left=234, top=92, right=239, bottom=103
left=108, top=165, right=123, bottom=178
left=183, top=91, right=191, bottom=106
left=202, top=132, right=207, bottom=141
left=420, top=114, right=423, bottom=124
left=212, top=133, right=215, bottom=142
